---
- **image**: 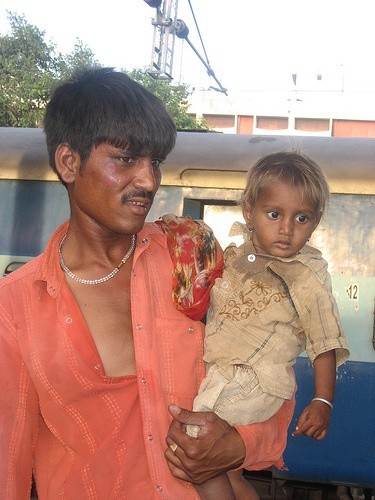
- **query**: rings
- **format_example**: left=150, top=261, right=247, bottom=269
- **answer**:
left=169, top=442, right=180, bottom=456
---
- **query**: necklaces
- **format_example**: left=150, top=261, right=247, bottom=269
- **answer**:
left=58, top=231, right=137, bottom=286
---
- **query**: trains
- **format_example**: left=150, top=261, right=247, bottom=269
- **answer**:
left=0, top=125, right=375, bottom=500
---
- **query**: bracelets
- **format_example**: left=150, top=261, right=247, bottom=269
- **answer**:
left=311, top=397, right=333, bottom=409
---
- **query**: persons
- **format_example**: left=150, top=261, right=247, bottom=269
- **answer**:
left=1, top=66, right=296, bottom=499
left=183, top=151, right=350, bottom=499
